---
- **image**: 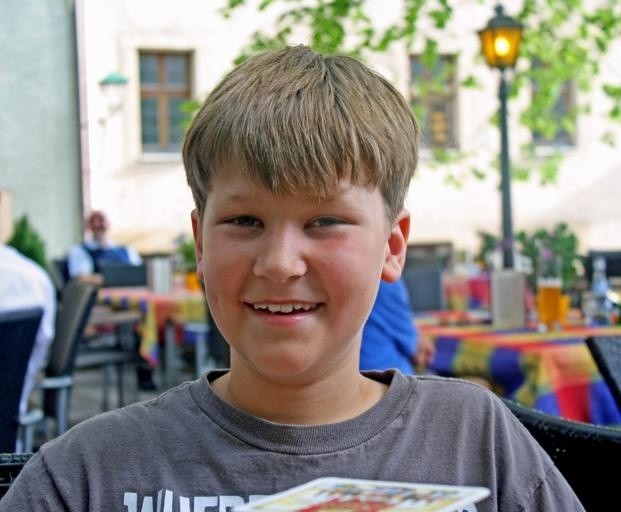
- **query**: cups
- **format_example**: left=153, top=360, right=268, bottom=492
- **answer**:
left=146, top=258, right=172, bottom=292
left=441, top=259, right=570, bottom=330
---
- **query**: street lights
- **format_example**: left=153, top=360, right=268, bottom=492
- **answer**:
left=476, top=2, right=526, bottom=269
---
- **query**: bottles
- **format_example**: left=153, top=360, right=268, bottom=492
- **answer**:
left=572, top=258, right=612, bottom=329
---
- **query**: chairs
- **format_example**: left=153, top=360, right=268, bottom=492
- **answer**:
left=0, top=274, right=103, bottom=452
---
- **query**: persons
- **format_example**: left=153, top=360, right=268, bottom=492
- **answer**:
left=357, top=264, right=438, bottom=380
left=0, top=185, right=59, bottom=448
left=0, top=46, right=585, bottom=512
left=68, top=208, right=156, bottom=391
